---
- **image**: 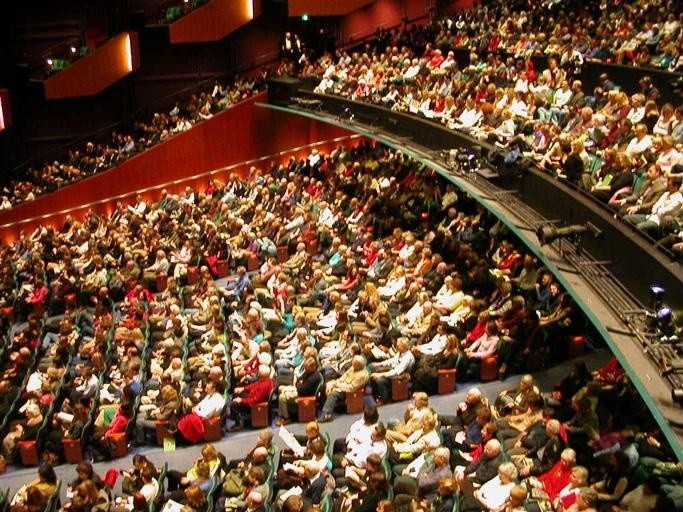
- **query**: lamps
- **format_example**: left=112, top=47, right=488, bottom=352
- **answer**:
left=647, top=284, right=682, bottom=412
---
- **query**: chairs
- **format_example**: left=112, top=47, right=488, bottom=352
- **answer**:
left=1, top=144, right=652, bottom=510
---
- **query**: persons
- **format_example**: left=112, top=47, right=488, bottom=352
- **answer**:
left=0, top=0, right=683, bottom=512
